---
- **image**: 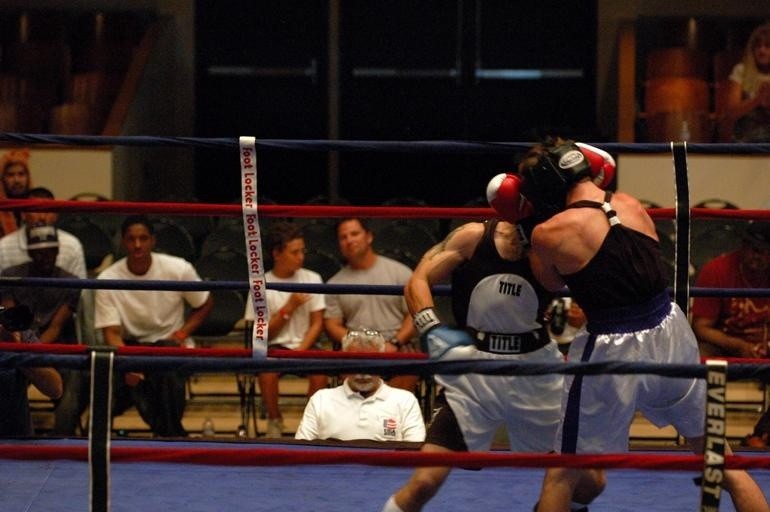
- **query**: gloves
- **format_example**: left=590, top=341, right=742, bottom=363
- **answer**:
left=416, top=307, right=477, bottom=381
left=486, top=173, right=534, bottom=221
left=575, top=141, right=617, bottom=191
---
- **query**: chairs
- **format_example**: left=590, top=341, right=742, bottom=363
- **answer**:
left=2, top=3, right=153, bottom=147
left=0, top=193, right=770, bottom=447
left=629, top=7, right=770, bottom=145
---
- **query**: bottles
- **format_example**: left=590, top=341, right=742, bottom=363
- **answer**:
left=200, top=415, right=215, bottom=440
left=235, top=425, right=248, bottom=438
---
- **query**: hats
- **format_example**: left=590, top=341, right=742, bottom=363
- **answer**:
left=23, top=223, right=60, bottom=250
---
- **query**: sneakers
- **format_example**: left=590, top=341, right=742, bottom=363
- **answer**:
left=265, top=418, right=285, bottom=439
left=20, top=364, right=64, bottom=400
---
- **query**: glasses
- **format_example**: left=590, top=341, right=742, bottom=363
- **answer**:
left=347, top=330, right=382, bottom=340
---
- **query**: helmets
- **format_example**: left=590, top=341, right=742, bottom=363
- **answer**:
left=520, top=137, right=593, bottom=208
